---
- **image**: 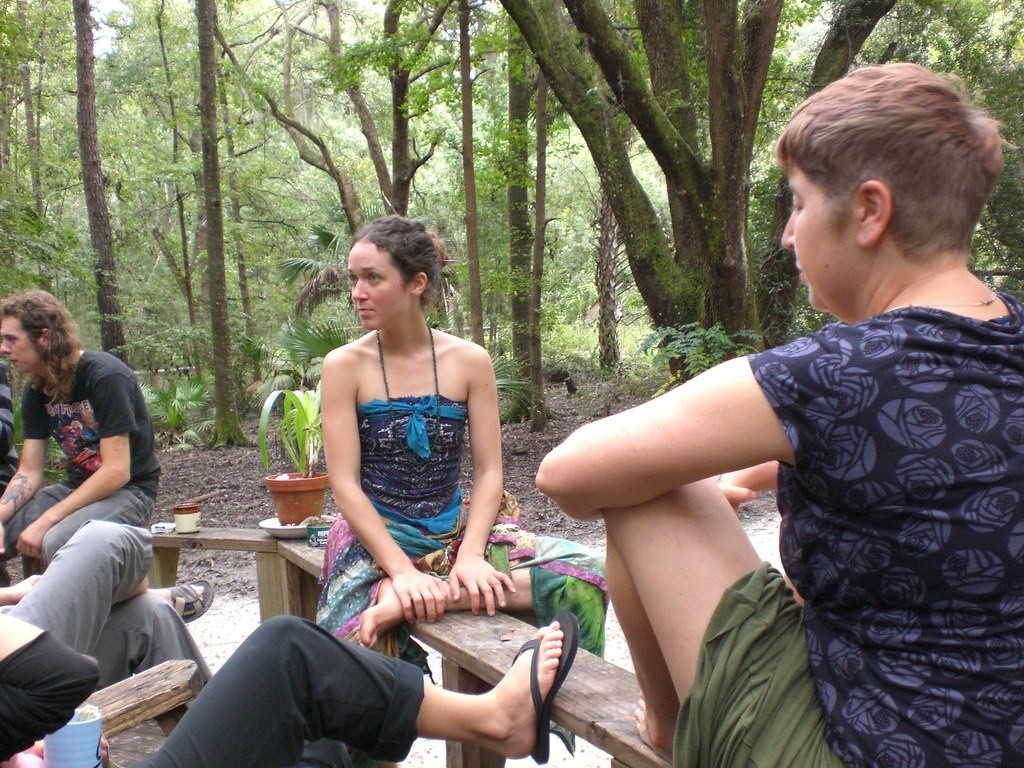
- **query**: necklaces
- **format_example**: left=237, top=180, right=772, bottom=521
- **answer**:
left=919, top=290, right=999, bottom=309
left=376, top=322, right=442, bottom=466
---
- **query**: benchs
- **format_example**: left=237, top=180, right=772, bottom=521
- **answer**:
left=277, top=540, right=673, bottom=768
left=21, top=526, right=276, bottom=624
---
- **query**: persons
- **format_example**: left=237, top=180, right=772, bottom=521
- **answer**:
left=0, top=290, right=161, bottom=581
left=314, top=215, right=610, bottom=768
left=0, top=610, right=580, bottom=768
left=532, top=63, right=1023, bottom=768
left=0, top=518, right=215, bottom=697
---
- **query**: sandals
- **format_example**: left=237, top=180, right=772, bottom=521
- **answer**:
left=166, top=578, right=216, bottom=625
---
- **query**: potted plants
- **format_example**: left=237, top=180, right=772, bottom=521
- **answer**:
left=257, top=378, right=330, bottom=525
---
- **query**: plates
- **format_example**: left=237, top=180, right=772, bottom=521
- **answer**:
left=258, top=515, right=341, bottom=539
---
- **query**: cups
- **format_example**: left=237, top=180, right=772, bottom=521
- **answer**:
left=43, top=709, right=103, bottom=768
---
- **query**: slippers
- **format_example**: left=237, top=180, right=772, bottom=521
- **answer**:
left=512, top=611, right=581, bottom=766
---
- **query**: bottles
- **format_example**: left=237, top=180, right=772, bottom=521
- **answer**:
left=174, top=504, right=202, bottom=533
